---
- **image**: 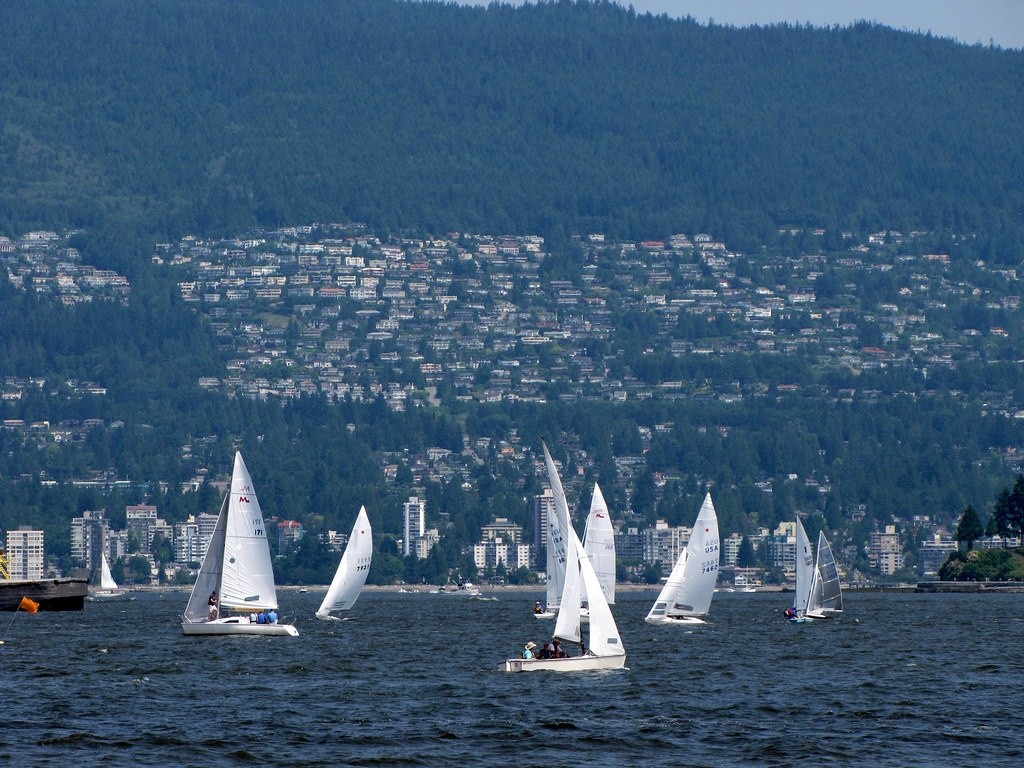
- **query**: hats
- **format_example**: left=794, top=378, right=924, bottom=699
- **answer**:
left=524, top=642, right=537, bottom=649
left=536, top=602, right=540, bottom=605
left=552, top=640, right=561, bottom=644
left=543, top=643, right=550, bottom=647
left=213, top=590, right=216, bottom=594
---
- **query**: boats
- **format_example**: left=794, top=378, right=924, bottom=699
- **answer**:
left=0, top=574, right=90, bottom=612
left=428, top=583, right=479, bottom=597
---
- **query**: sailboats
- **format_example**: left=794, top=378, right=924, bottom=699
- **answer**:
left=178, top=447, right=300, bottom=637
left=314, top=504, right=373, bottom=623
left=90, top=551, right=129, bottom=602
left=644, top=491, right=721, bottom=626
left=496, top=437, right=629, bottom=672
left=803, top=529, right=845, bottom=619
left=780, top=514, right=815, bottom=625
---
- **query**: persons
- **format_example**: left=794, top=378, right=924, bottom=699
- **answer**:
left=783, top=607, right=802, bottom=620
left=669, top=615, right=684, bottom=620
left=522, top=640, right=571, bottom=659
left=207, top=591, right=218, bottom=621
left=534, top=602, right=543, bottom=614
left=250, top=609, right=279, bottom=625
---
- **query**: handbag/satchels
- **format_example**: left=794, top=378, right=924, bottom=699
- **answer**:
left=264, top=617, right=269, bottom=624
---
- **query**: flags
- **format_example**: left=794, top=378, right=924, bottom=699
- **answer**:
left=20, top=597, right=39, bottom=614
left=0, top=554, right=11, bottom=580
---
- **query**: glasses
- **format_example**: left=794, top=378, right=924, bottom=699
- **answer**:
left=536, top=606, right=539, bottom=607
